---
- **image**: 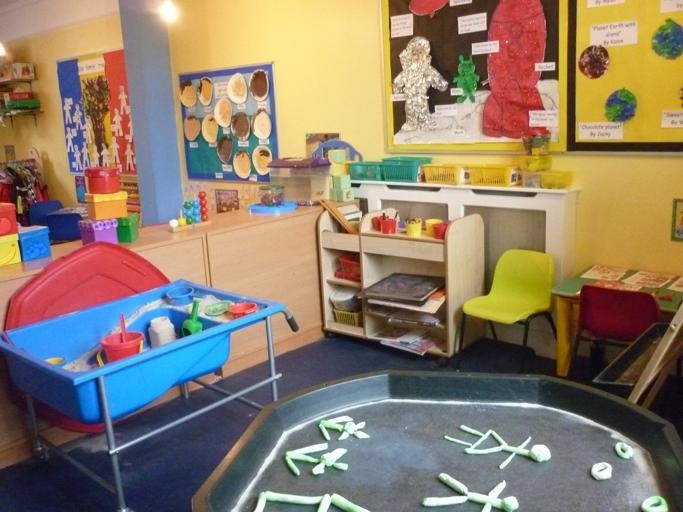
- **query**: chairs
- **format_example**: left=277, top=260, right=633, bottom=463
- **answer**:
left=578, top=284, right=666, bottom=374
left=459, top=248, right=555, bottom=371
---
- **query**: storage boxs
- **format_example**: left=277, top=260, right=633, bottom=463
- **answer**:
left=266, top=158, right=331, bottom=202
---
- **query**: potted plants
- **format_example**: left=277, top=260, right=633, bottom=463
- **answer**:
left=75, top=75, right=120, bottom=194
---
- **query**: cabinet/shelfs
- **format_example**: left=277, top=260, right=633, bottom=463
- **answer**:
left=0, top=278, right=299, bottom=512
left=0, top=221, right=221, bottom=475
left=0, top=61, right=44, bottom=128
left=316, top=206, right=484, bottom=374
left=204, top=184, right=361, bottom=392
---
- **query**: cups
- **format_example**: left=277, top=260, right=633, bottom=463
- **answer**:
left=373, top=215, right=396, bottom=234
left=425, top=217, right=447, bottom=239
left=403, top=217, right=421, bottom=235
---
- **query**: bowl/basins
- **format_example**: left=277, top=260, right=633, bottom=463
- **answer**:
left=165, top=286, right=194, bottom=308
left=228, top=302, right=256, bottom=319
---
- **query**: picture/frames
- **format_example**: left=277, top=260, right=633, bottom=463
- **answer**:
left=671, top=199, right=683, bottom=239
left=381, top=0, right=568, bottom=155
left=169, top=61, right=280, bottom=182
left=567, top=0, right=683, bottom=153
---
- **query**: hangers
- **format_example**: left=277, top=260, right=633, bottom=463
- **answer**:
left=0, top=158, right=36, bottom=175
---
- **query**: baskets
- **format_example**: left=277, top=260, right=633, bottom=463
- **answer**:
left=333, top=308, right=363, bottom=327
left=348, top=157, right=573, bottom=190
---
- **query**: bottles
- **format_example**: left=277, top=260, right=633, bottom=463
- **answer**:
left=146, top=316, right=176, bottom=349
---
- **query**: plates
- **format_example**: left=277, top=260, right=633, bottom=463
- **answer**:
left=176, top=68, right=274, bottom=180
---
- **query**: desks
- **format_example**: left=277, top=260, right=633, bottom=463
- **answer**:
left=328, top=173, right=582, bottom=366
left=548, top=261, right=683, bottom=385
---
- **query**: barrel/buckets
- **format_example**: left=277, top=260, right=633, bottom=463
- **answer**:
left=96, top=314, right=145, bottom=368
left=84, top=166, right=123, bottom=194
